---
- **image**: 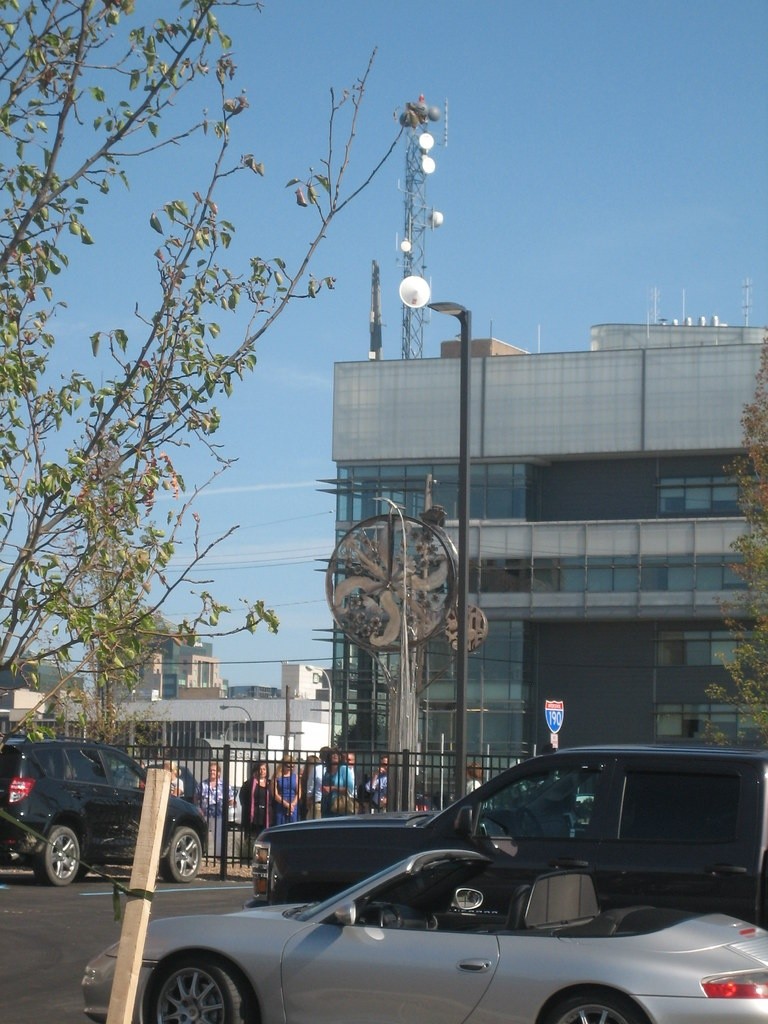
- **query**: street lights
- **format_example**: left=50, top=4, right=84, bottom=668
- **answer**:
left=427, top=299, right=473, bottom=801
left=220, top=704, right=253, bottom=780
left=374, top=495, right=410, bottom=751
left=305, top=666, right=332, bottom=749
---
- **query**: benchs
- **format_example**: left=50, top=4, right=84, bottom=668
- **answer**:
left=571, top=904, right=657, bottom=941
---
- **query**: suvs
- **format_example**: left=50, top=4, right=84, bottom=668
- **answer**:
left=0, top=733, right=209, bottom=885
left=245, top=744, right=768, bottom=927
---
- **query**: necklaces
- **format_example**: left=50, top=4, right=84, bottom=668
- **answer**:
left=260, top=778, right=266, bottom=782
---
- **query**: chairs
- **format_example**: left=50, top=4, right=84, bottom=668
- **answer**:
left=503, top=883, right=532, bottom=931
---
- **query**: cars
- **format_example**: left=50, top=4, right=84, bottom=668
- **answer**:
left=228, top=787, right=242, bottom=829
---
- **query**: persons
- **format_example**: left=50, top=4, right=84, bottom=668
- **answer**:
left=195, top=762, right=234, bottom=862
left=320, top=749, right=353, bottom=815
left=239, top=762, right=272, bottom=858
left=271, top=755, right=301, bottom=824
left=139, top=761, right=184, bottom=796
left=306, top=747, right=331, bottom=819
left=357, top=774, right=369, bottom=814
left=345, top=752, right=364, bottom=814
left=467, top=762, right=483, bottom=794
left=365, top=756, right=388, bottom=813
left=302, top=755, right=319, bottom=820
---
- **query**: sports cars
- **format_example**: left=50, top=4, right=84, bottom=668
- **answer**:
left=82, top=849, right=768, bottom=1024
